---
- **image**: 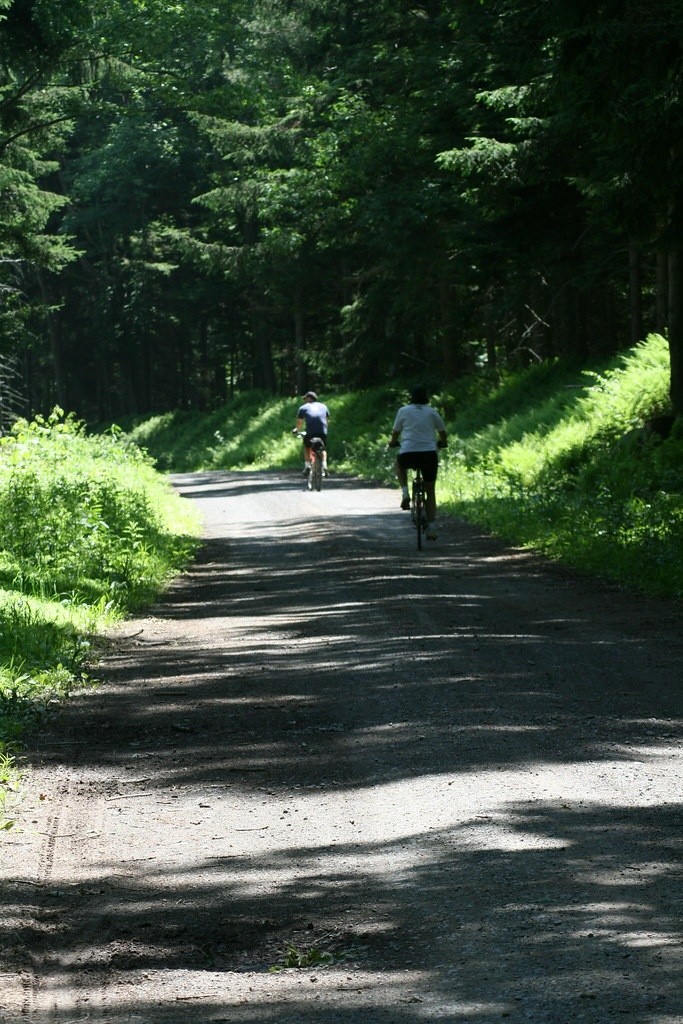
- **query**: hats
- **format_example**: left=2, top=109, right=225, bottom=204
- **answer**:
left=303, top=391, right=318, bottom=399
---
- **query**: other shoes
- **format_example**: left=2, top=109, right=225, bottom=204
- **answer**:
left=401, top=495, right=410, bottom=510
left=322, top=468, right=329, bottom=478
left=303, top=466, right=311, bottom=476
left=427, top=529, right=439, bottom=540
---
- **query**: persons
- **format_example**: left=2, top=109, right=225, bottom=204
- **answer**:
left=293, top=391, right=332, bottom=478
left=388, top=385, right=448, bottom=540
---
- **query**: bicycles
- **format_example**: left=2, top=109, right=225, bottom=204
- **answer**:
left=393, top=443, right=442, bottom=551
left=296, top=431, right=325, bottom=492
